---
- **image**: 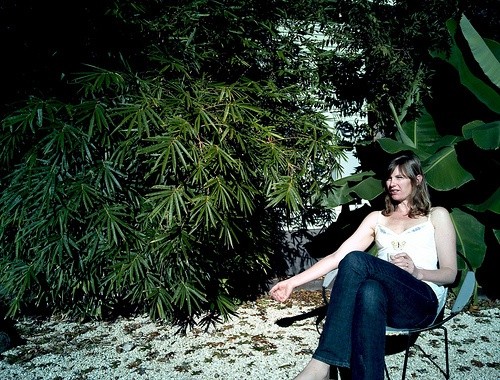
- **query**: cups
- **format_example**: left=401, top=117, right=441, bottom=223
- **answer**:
left=387, top=252, right=407, bottom=267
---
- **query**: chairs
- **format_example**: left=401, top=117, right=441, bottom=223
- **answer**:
left=321, top=250, right=476, bottom=379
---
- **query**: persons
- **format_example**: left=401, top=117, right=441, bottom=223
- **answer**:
left=267, top=153, right=457, bottom=380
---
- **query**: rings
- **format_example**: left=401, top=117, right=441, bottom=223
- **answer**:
left=406, top=262, right=409, bottom=267
